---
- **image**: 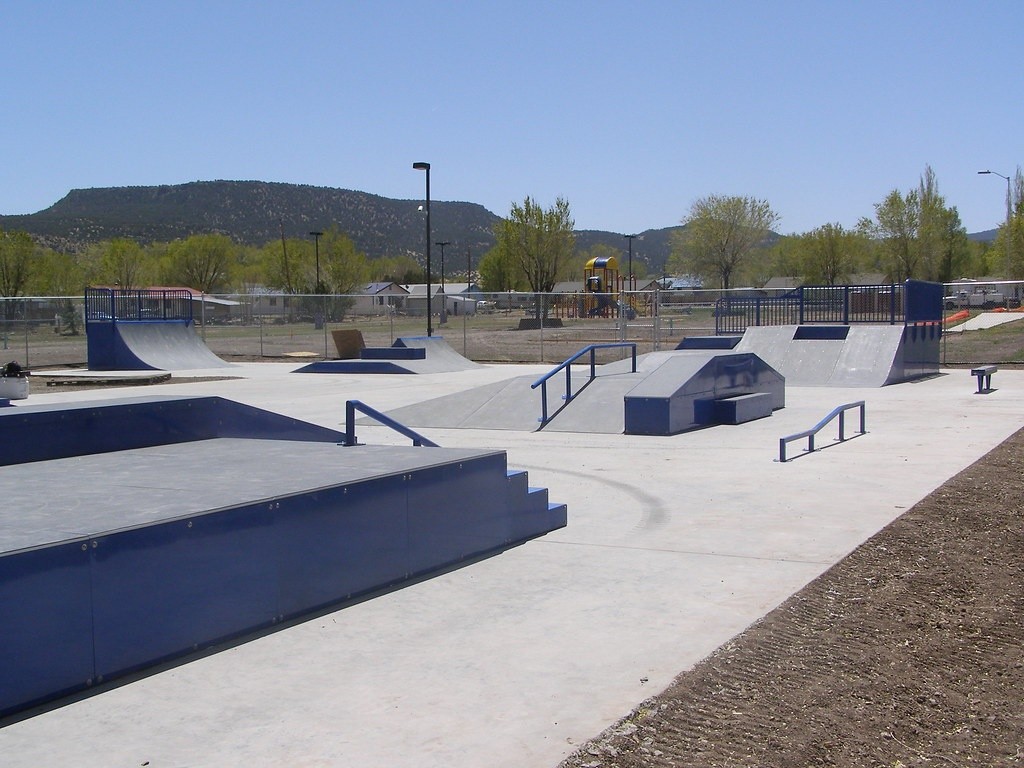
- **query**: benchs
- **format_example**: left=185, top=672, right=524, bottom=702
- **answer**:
left=971, top=365, right=997, bottom=391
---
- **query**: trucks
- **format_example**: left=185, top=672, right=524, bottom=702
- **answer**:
left=943, top=290, right=1005, bottom=310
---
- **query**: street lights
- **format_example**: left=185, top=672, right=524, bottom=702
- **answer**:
left=415, top=163, right=433, bottom=340
left=435, top=241, right=450, bottom=313
left=625, top=235, right=637, bottom=291
left=309, top=232, right=324, bottom=312
left=977, top=171, right=1010, bottom=312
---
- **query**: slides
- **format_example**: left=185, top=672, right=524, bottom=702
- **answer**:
left=589, top=292, right=634, bottom=320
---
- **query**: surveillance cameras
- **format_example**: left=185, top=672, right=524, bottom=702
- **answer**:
left=418, top=206, right=423, bottom=212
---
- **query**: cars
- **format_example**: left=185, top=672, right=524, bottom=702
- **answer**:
left=138, top=309, right=154, bottom=317
left=87, top=313, right=118, bottom=320
left=1005, top=298, right=1021, bottom=307
left=477, top=301, right=488, bottom=308
